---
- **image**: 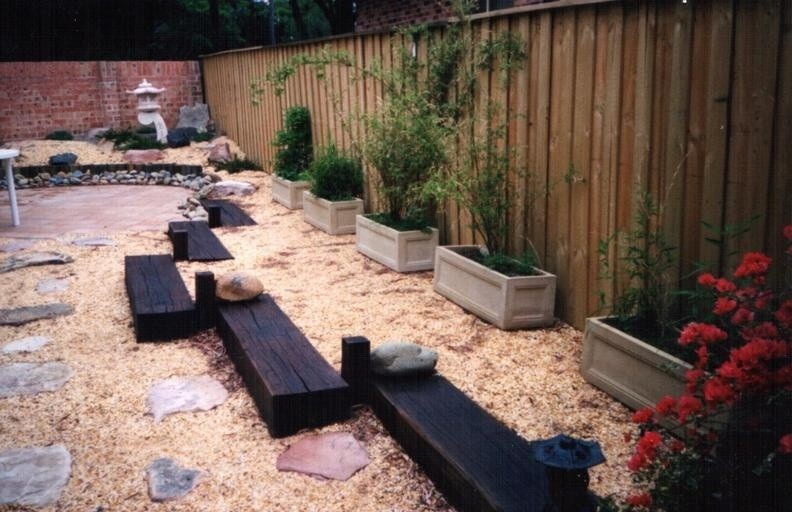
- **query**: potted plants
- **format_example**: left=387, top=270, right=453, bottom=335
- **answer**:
left=269, top=104, right=315, bottom=210
left=578, top=183, right=791, bottom=454
left=307, top=26, right=477, bottom=276
left=298, top=133, right=365, bottom=235
left=249, top=3, right=558, bottom=331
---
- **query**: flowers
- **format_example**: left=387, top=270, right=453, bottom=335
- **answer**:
left=623, top=223, right=791, bottom=512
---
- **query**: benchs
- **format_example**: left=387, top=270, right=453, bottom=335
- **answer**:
left=122, top=256, right=201, bottom=342
left=211, top=291, right=353, bottom=437
left=365, top=369, right=599, bottom=512
